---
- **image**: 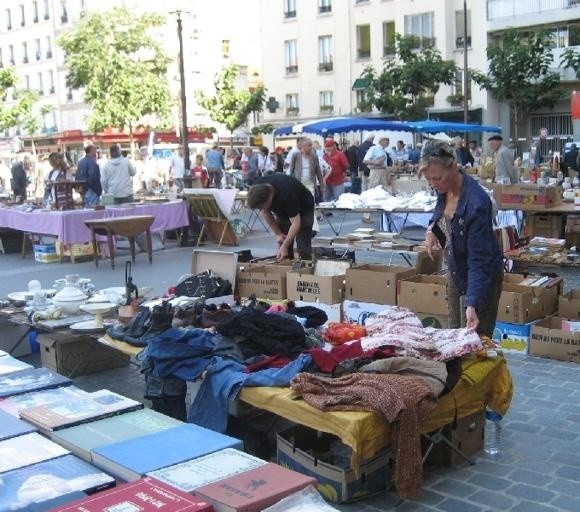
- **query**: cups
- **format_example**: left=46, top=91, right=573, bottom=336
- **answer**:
left=54, top=273, right=94, bottom=294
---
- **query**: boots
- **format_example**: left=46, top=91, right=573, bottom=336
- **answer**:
left=107, top=297, right=232, bottom=347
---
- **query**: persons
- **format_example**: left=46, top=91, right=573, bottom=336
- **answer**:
left=247, top=168, right=317, bottom=262
left=560, top=143, right=580, bottom=180
left=488, top=136, right=521, bottom=183
left=415, top=138, right=505, bottom=339
left=0, top=134, right=485, bottom=222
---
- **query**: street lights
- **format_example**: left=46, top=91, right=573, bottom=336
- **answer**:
left=169, top=7, right=193, bottom=187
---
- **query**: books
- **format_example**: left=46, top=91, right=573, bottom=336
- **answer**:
left=518, top=233, right=567, bottom=263
left=315, top=226, right=430, bottom=254
left=0, top=349, right=320, bottom=512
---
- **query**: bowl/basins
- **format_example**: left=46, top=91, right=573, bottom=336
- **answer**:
left=6, top=288, right=148, bottom=322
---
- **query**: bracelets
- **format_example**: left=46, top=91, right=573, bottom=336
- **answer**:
left=425, top=228, right=433, bottom=234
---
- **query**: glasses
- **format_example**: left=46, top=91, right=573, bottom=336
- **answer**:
left=419, top=148, right=455, bottom=160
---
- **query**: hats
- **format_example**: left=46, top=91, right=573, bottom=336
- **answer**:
left=488, top=134, right=502, bottom=141
left=378, top=135, right=389, bottom=142
left=324, top=140, right=335, bottom=148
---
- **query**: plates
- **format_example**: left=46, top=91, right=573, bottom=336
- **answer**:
left=69, top=321, right=111, bottom=332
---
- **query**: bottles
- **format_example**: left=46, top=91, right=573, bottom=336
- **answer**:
left=537, top=168, right=580, bottom=201
left=229, top=213, right=244, bottom=234
left=482, top=407, right=504, bottom=455
left=154, top=181, right=167, bottom=195
